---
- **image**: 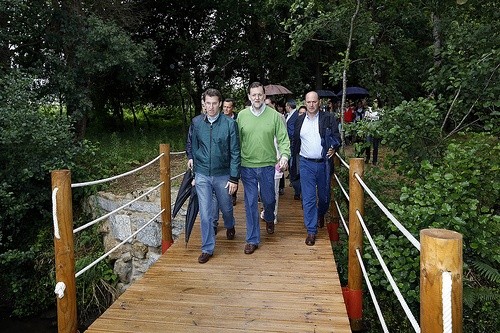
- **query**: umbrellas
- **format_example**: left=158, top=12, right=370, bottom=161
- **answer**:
left=316, top=90, right=337, bottom=100
left=263, top=84, right=293, bottom=96
left=171, top=163, right=199, bottom=249
left=336, top=87, right=371, bottom=97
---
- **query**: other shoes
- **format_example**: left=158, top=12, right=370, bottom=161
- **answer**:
left=286, top=174, right=290, bottom=179
left=214, top=223, right=218, bottom=235
left=365, top=160, right=369, bottom=164
left=289, top=183, right=293, bottom=187
left=373, top=162, right=377, bottom=165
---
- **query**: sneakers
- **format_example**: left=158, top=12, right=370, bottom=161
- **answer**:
left=279, top=187, right=284, bottom=195
left=233, top=197, right=236, bottom=206
left=261, top=211, right=278, bottom=224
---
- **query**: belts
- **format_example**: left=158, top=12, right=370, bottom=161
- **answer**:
left=300, top=155, right=324, bottom=163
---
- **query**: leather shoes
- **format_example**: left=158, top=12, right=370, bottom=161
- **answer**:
left=226, top=226, right=235, bottom=240
left=198, top=253, right=213, bottom=263
left=305, top=234, right=315, bottom=246
left=318, top=216, right=324, bottom=228
left=266, top=220, right=274, bottom=234
left=294, top=194, right=300, bottom=200
left=244, top=244, right=257, bottom=254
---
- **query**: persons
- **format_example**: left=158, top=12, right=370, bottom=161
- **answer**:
left=186, top=82, right=382, bottom=264
